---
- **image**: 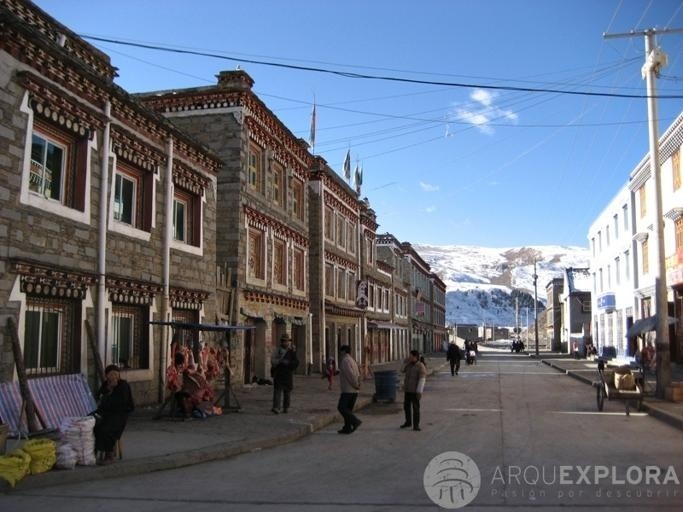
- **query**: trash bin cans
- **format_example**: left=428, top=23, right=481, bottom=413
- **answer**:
left=373, top=370, right=397, bottom=402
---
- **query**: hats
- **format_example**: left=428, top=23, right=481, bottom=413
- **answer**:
left=280, top=334, right=293, bottom=342
left=105, top=365, right=120, bottom=376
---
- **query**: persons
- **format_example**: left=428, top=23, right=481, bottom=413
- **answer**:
left=88, top=365, right=134, bottom=465
left=511, top=340, right=525, bottom=353
left=446, top=341, right=462, bottom=376
left=573, top=341, right=581, bottom=360
left=465, top=339, right=477, bottom=365
left=338, top=344, right=362, bottom=434
left=271, top=334, right=298, bottom=414
left=401, top=350, right=426, bottom=431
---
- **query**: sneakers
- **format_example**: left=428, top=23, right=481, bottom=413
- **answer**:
left=96, top=457, right=114, bottom=465
left=271, top=407, right=288, bottom=415
left=337, top=420, right=362, bottom=435
left=400, top=422, right=422, bottom=431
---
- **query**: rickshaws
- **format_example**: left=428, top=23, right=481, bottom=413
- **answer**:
left=596, top=368, right=643, bottom=413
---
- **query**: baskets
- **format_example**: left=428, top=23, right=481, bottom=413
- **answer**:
left=614, top=367, right=635, bottom=389
